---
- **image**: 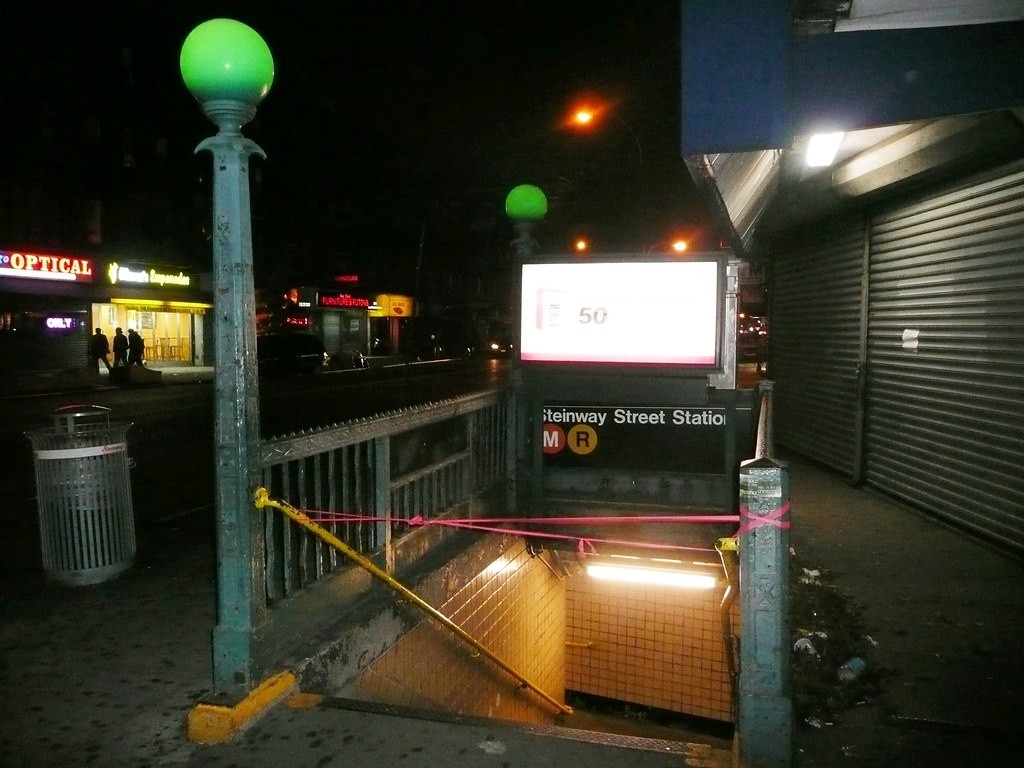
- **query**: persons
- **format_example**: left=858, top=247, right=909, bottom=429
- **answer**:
left=91, top=327, right=112, bottom=374
left=128, top=328, right=145, bottom=365
left=113, top=327, right=129, bottom=367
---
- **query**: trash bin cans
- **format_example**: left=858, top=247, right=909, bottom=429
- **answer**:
left=24, top=402, right=138, bottom=586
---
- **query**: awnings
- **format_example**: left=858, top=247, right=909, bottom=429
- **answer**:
left=679, top=0, right=1023, bottom=258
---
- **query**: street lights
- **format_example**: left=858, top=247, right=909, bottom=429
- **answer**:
left=568, top=97, right=647, bottom=252
